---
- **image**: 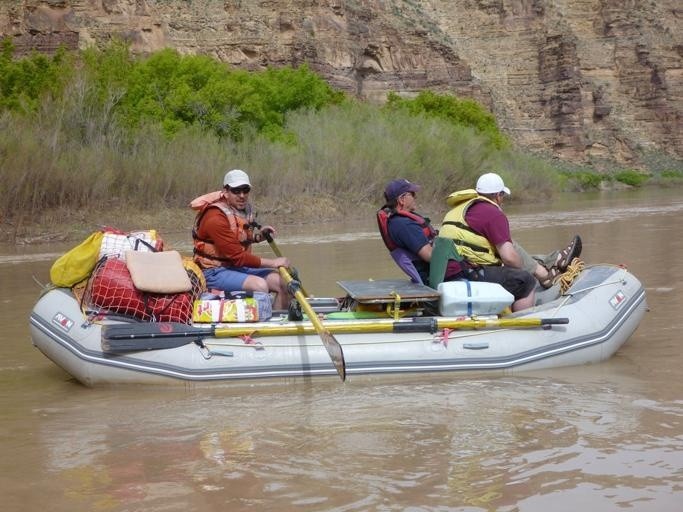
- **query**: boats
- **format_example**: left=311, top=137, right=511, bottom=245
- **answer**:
left=29, top=259, right=649, bottom=387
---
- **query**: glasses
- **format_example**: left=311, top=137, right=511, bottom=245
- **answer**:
left=227, top=185, right=250, bottom=193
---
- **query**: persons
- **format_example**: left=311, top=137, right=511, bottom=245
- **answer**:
left=440, top=170, right=535, bottom=313
left=192, top=168, right=292, bottom=311
left=384, top=179, right=582, bottom=289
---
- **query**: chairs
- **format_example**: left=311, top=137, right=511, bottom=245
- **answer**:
left=375, top=207, right=423, bottom=286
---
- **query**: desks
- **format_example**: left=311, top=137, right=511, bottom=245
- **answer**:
left=336, top=277, right=441, bottom=317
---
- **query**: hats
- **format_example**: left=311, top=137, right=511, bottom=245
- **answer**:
left=384, top=178, right=421, bottom=202
left=223, top=168, right=252, bottom=188
left=476, top=172, right=511, bottom=195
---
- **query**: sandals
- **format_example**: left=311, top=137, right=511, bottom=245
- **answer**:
left=556, top=234, right=583, bottom=271
left=539, top=267, right=560, bottom=289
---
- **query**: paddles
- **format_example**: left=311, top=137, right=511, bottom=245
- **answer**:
left=99, top=318, right=573, bottom=349
left=278, top=266, right=346, bottom=381
